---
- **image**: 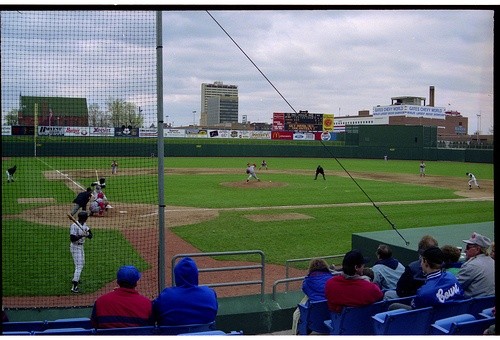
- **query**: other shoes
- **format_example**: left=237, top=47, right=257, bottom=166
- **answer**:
left=71, top=282, right=81, bottom=292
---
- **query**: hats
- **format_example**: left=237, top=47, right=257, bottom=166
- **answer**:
left=462, top=232, right=490, bottom=249
left=343, top=248, right=370, bottom=266
left=117, top=265, right=140, bottom=285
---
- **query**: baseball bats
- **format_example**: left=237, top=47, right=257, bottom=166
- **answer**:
left=66, top=212, right=89, bottom=235
left=96, top=169, right=99, bottom=187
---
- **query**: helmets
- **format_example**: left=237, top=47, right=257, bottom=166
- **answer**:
left=99, top=178, right=106, bottom=183
left=98, top=193, right=104, bottom=198
left=78, top=211, right=89, bottom=218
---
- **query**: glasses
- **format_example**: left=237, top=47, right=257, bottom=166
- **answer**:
left=466, top=244, right=473, bottom=250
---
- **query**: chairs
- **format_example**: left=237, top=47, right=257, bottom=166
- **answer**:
left=1, top=317, right=244, bottom=336
left=296, top=286, right=494, bottom=335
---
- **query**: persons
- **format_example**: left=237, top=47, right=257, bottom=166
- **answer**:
left=246, top=162, right=261, bottom=182
left=466, top=172, right=481, bottom=190
left=420, top=161, right=427, bottom=177
left=111, top=159, right=117, bottom=175
left=151, top=256, right=219, bottom=336
left=69, top=211, right=92, bottom=293
left=70, top=177, right=111, bottom=220
left=259, top=160, right=268, bottom=171
left=6, top=165, right=17, bottom=183
left=314, top=166, right=327, bottom=181
left=90, top=266, right=156, bottom=330
left=292, top=231, right=499, bottom=335
